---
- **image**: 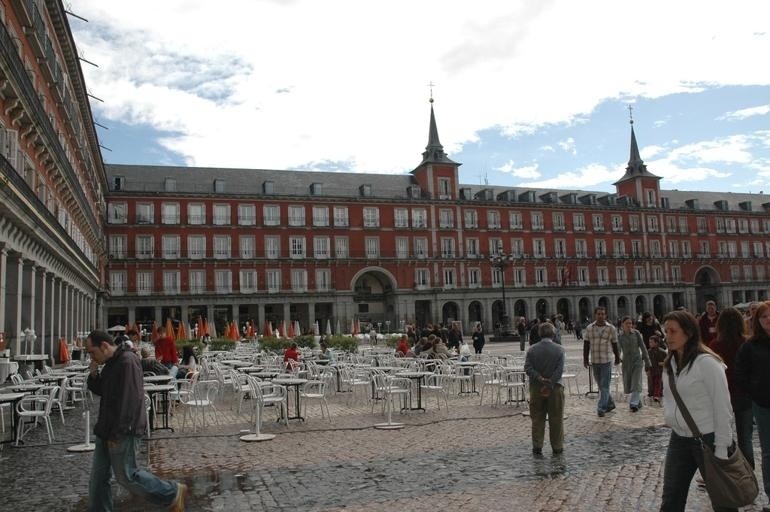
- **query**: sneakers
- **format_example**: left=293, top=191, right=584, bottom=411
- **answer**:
left=169, top=483, right=188, bottom=512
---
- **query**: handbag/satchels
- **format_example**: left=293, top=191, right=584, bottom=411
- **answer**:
left=693, top=436, right=760, bottom=510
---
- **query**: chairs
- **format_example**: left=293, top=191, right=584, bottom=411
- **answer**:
left=0, top=320, right=628, bottom=453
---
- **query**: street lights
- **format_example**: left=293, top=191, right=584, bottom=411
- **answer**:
left=488, top=246, right=521, bottom=343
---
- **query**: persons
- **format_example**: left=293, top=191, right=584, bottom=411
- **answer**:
left=243, top=321, right=251, bottom=339
left=283, top=342, right=298, bottom=370
left=524, top=322, right=566, bottom=454
left=85, top=331, right=188, bottom=512
left=72, top=336, right=85, bottom=360
left=200, top=333, right=210, bottom=346
left=370, top=327, right=377, bottom=344
left=472, top=326, right=485, bottom=358
left=582, top=298, right=770, bottom=512
left=114, top=326, right=198, bottom=411
left=518, top=313, right=582, bottom=351
left=394, top=321, right=463, bottom=359
left=319, top=334, right=331, bottom=353
left=494, top=320, right=501, bottom=337
